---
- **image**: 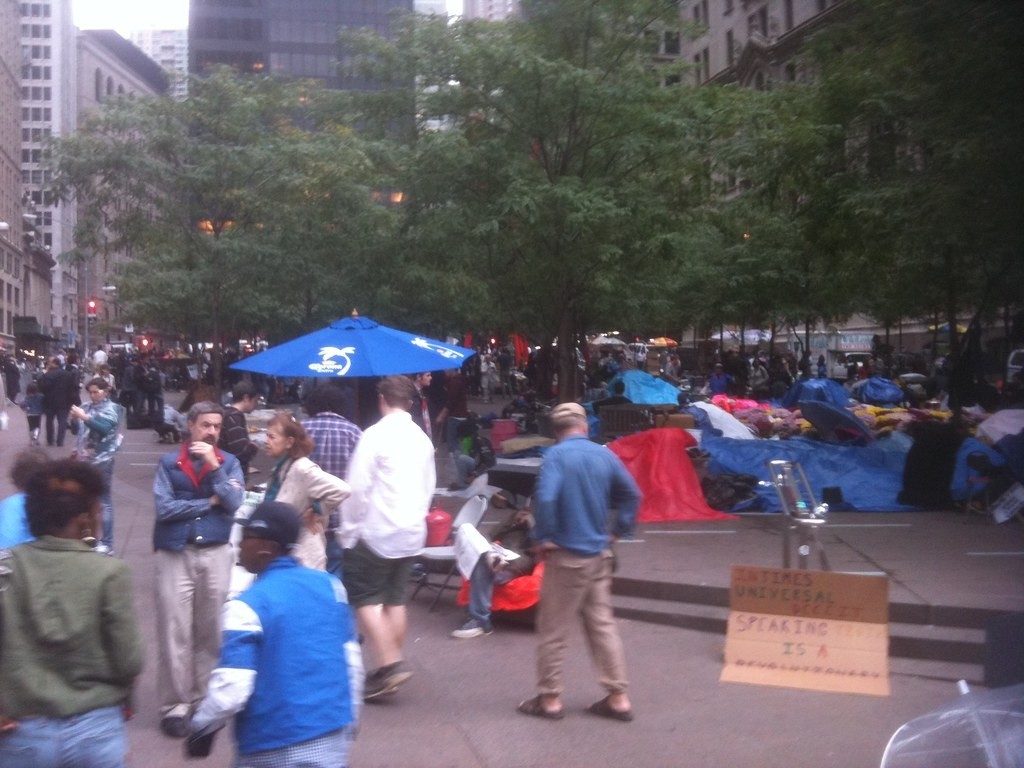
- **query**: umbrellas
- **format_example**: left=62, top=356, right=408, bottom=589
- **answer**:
left=228, top=307, right=478, bottom=379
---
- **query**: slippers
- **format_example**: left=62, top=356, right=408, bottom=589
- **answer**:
left=517, top=689, right=568, bottom=723
left=587, top=692, right=638, bottom=722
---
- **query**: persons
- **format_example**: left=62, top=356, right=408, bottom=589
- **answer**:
left=260, top=413, right=353, bottom=573
left=0, top=446, right=54, bottom=551
left=1, top=331, right=1024, bottom=493
left=150, top=401, right=246, bottom=736
left=67, top=378, right=121, bottom=557
left=517, top=401, right=643, bottom=723
left=332, top=374, right=437, bottom=701
left=297, top=379, right=363, bottom=584
left=0, top=459, right=146, bottom=768
left=181, top=502, right=368, bottom=768
left=451, top=500, right=546, bottom=640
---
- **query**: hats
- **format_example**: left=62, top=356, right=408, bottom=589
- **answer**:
left=226, top=498, right=300, bottom=549
left=551, top=402, right=589, bottom=419
left=714, top=363, right=723, bottom=369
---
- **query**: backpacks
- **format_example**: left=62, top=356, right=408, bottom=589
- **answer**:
left=143, top=371, right=162, bottom=396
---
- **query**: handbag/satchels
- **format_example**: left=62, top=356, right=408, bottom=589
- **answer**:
left=818, top=364, right=827, bottom=376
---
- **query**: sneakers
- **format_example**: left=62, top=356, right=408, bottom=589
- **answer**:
left=453, top=613, right=495, bottom=642
left=91, top=542, right=115, bottom=558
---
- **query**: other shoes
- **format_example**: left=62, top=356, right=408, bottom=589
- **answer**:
left=161, top=695, right=197, bottom=737
left=361, top=661, right=415, bottom=701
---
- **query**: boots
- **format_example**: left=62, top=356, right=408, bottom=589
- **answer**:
left=29, top=429, right=36, bottom=448
left=33, top=426, right=41, bottom=448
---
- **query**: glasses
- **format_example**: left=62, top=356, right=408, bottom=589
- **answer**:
left=88, top=389, right=106, bottom=397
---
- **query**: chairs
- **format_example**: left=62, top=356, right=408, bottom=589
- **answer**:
left=412, top=491, right=490, bottom=611
